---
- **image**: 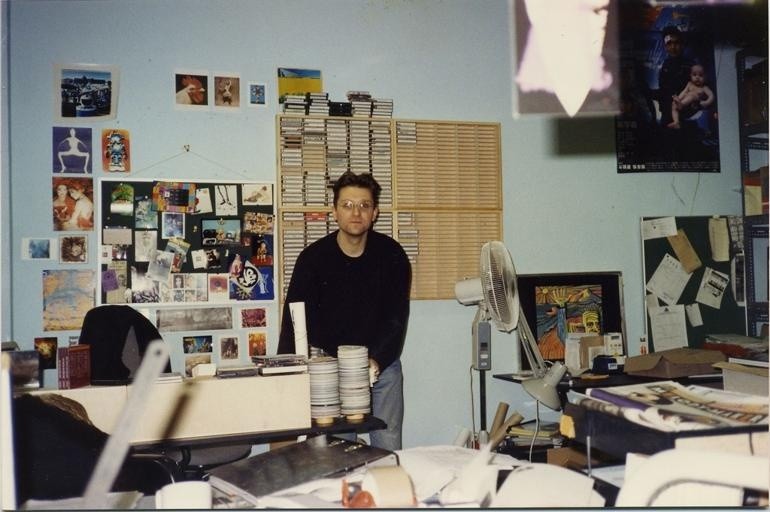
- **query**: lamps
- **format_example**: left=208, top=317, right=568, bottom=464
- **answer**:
left=517, top=308, right=568, bottom=411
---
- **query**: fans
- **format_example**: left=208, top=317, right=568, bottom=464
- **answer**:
left=454, top=241, right=519, bottom=453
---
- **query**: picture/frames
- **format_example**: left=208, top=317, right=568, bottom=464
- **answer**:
left=59, top=235, right=89, bottom=264
left=161, top=212, right=186, bottom=240
left=246, top=80, right=269, bottom=109
left=275, top=66, right=324, bottom=115
left=213, top=184, right=240, bottom=218
left=202, top=218, right=243, bottom=248
left=516, top=270, right=628, bottom=377
left=171, top=73, right=211, bottom=112
left=212, top=74, right=243, bottom=112
left=52, top=64, right=119, bottom=124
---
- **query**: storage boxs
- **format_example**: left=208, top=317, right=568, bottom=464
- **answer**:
left=711, top=359, right=768, bottom=399
left=624, top=347, right=727, bottom=379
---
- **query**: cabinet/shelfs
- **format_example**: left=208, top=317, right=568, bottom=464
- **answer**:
left=735, top=34, right=768, bottom=341
left=561, top=386, right=768, bottom=477
left=275, top=114, right=504, bottom=301
left=506, top=434, right=570, bottom=463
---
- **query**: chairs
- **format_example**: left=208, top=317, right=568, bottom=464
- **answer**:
left=78, top=306, right=251, bottom=494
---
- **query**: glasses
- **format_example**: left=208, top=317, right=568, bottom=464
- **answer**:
left=334, top=197, right=377, bottom=210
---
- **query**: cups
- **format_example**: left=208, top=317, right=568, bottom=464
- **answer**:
left=154, top=480, right=213, bottom=511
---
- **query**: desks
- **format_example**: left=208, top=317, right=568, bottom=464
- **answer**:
left=493, top=366, right=724, bottom=390
left=131, top=411, right=387, bottom=455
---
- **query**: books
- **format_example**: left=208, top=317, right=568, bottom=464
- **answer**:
left=205, top=432, right=399, bottom=507
left=57, top=344, right=92, bottom=388
left=258, top=364, right=308, bottom=375
left=276, top=95, right=396, bottom=170
left=157, top=371, right=183, bottom=385
left=728, top=353, right=770, bottom=368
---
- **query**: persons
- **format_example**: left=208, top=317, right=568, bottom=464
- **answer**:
left=240, top=238, right=252, bottom=263
left=244, top=186, right=267, bottom=202
left=222, top=80, right=232, bottom=106
left=258, top=241, right=267, bottom=255
left=260, top=217, right=273, bottom=234
left=278, top=172, right=411, bottom=451
left=174, top=276, right=181, bottom=289
left=659, top=26, right=696, bottom=133
left=68, top=181, right=93, bottom=230
left=670, top=65, right=715, bottom=128
left=51, top=180, right=74, bottom=230
